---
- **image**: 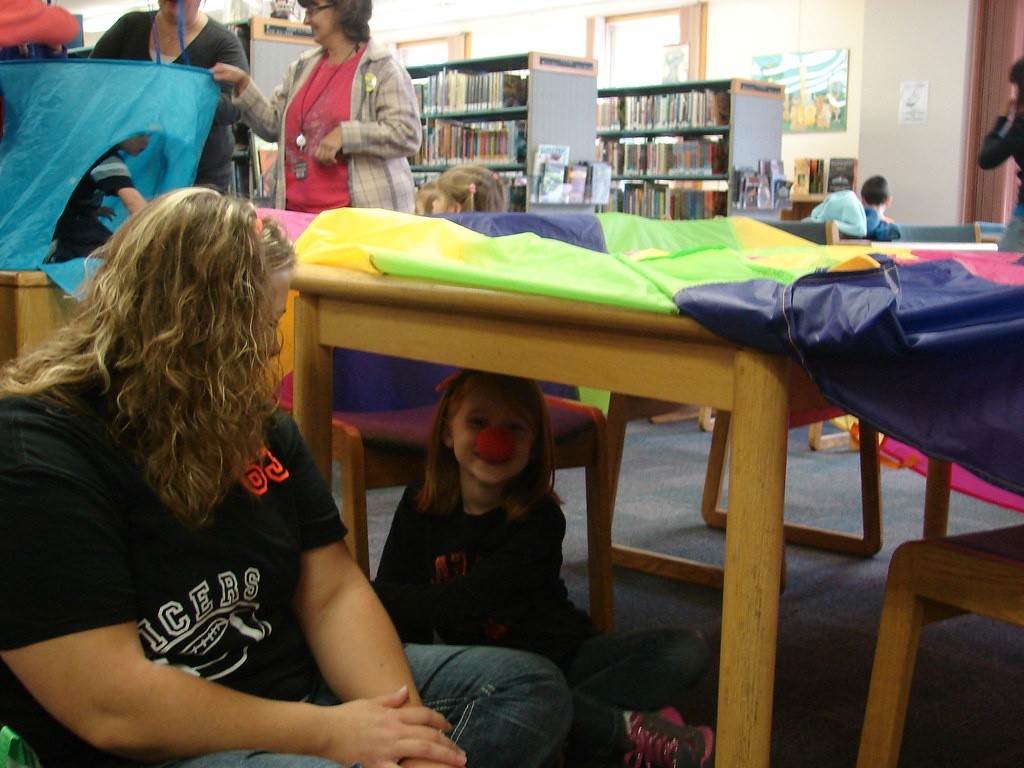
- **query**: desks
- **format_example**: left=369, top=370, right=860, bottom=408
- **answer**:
left=288, top=209, right=1023, bottom=768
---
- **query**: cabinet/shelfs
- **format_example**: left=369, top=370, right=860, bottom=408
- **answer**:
left=597, top=79, right=784, bottom=220
left=225, top=17, right=323, bottom=207
left=405, top=52, right=598, bottom=212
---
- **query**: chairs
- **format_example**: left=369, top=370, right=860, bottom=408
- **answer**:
left=606, top=391, right=884, bottom=593
left=700, top=214, right=851, bottom=450
left=856, top=523, right=1024, bottom=768
left=256, top=208, right=615, bottom=636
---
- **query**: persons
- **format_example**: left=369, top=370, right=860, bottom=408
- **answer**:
left=372, top=368, right=712, bottom=768
left=208, top=0, right=421, bottom=216
left=411, top=162, right=507, bottom=217
left=39, top=134, right=153, bottom=263
left=858, top=176, right=901, bottom=241
left=0, top=187, right=574, bottom=768
left=0, top=44, right=68, bottom=70
left=89, top=0, right=248, bottom=196
left=977, top=56, right=1024, bottom=253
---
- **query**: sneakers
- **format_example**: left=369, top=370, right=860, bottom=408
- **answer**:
left=620, top=708, right=713, bottom=768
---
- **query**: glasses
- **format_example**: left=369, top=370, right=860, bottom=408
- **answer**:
left=305, top=5, right=333, bottom=16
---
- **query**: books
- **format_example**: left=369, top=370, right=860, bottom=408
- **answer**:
left=229, top=126, right=278, bottom=199
left=662, top=43, right=690, bottom=85
left=407, top=67, right=527, bottom=211
left=530, top=90, right=857, bottom=221
